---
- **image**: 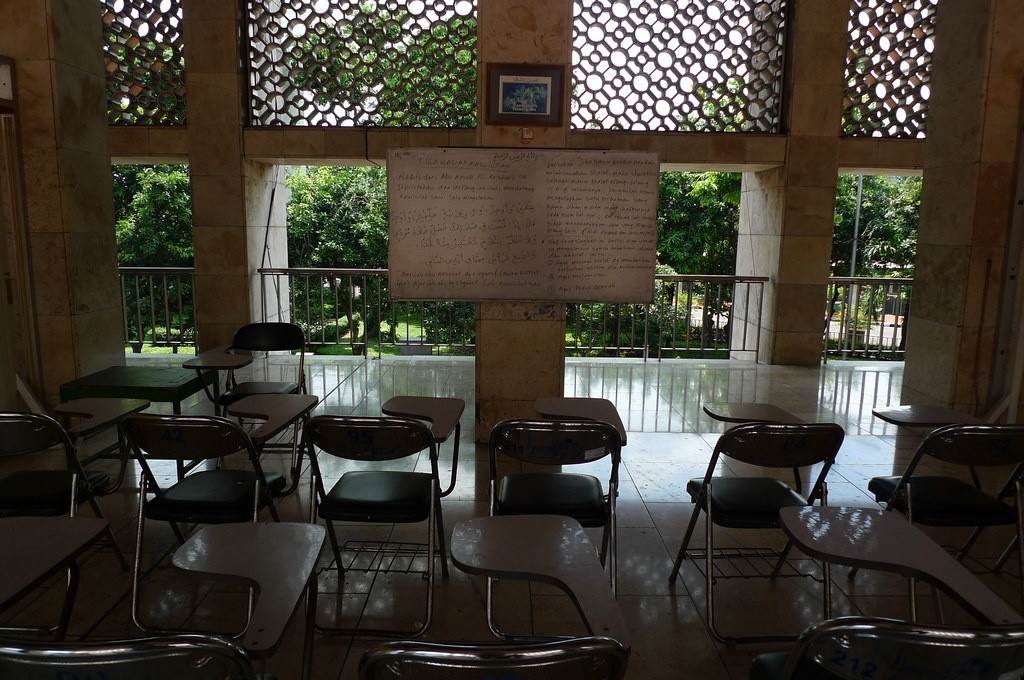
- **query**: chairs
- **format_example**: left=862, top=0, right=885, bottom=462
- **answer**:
left=0, top=322, right=1024, bottom=680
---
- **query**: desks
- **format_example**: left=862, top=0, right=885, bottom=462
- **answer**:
left=59, top=365, right=220, bottom=482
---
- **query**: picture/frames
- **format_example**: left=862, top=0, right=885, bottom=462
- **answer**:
left=485, top=62, right=566, bottom=128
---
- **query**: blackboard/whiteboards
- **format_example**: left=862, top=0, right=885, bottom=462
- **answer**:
left=382, top=144, right=660, bottom=305
